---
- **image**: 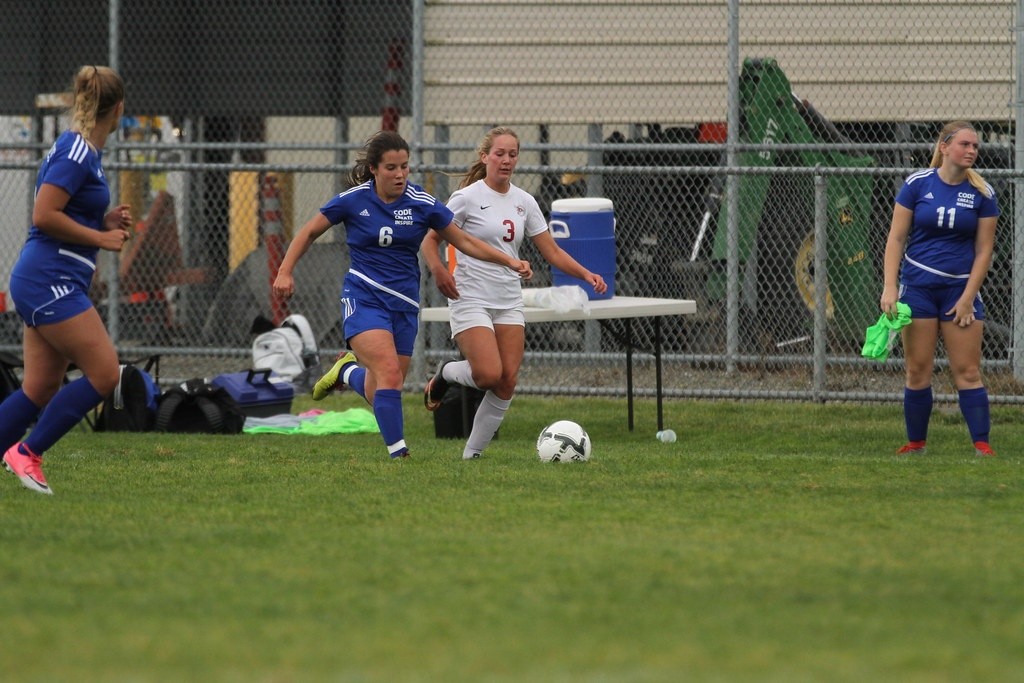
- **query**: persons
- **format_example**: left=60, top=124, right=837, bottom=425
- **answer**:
left=879, top=119, right=999, bottom=459
left=272, top=132, right=534, bottom=463
left=420, top=126, right=609, bottom=460
left=0, top=66, right=134, bottom=497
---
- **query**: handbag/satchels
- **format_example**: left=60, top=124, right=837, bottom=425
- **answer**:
left=157, top=378, right=246, bottom=433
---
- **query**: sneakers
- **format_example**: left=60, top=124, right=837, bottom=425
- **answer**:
left=393, top=451, right=416, bottom=461
left=1, top=442, right=53, bottom=495
left=312, top=351, right=358, bottom=401
left=974, top=440, right=995, bottom=458
left=899, top=442, right=926, bottom=456
left=425, top=357, right=456, bottom=411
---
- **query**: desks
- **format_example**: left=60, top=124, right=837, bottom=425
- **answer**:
left=421, top=290, right=696, bottom=433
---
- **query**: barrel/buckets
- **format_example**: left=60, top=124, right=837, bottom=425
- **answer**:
left=548, top=198, right=617, bottom=301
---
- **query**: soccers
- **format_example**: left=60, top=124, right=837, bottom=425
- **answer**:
left=536, top=420, right=592, bottom=464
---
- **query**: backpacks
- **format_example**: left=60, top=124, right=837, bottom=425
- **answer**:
left=251, top=314, right=317, bottom=390
left=96, top=364, right=159, bottom=432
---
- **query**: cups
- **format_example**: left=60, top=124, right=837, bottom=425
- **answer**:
left=655, top=429, right=677, bottom=443
